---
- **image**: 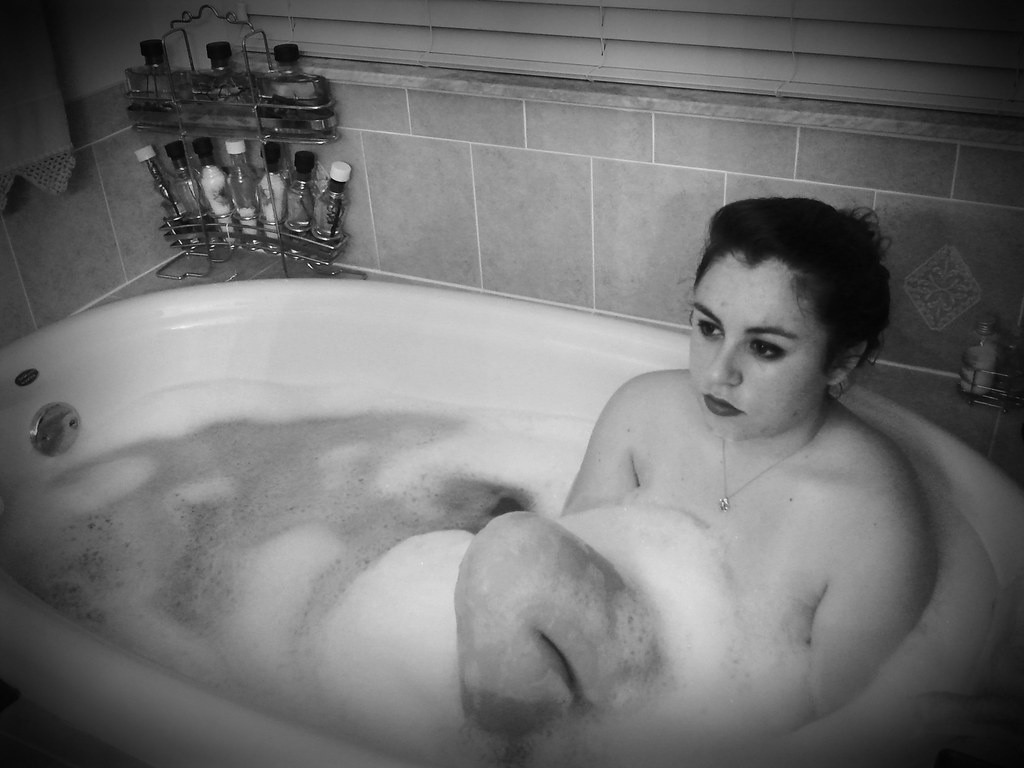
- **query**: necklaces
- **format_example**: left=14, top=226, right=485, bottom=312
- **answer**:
left=719, top=408, right=830, bottom=511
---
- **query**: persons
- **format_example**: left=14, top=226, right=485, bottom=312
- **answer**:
left=454, top=197, right=933, bottom=734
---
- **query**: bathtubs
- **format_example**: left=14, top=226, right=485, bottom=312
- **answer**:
left=1, top=244, right=1024, bottom=768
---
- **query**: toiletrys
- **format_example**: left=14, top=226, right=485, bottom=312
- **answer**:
left=134, top=139, right=353, bottom=255
left=124, top=38, right=340, bottom=134
left=956, top=311, right=1002, bottom=394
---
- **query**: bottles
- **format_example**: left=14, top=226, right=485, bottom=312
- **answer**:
left=225, top=139, right=258, bottom=236
left=287, top=151, right=317, bottom=232
left=960, top=314, right=999, bottom=395
left=160, top=140, right=202, bottom=212
left=136, top=145, right=185, bottom=215
left=191, top=136, right=235, bottom=242
left=312, top=161, right=351, bottom=241
left=256, top=141, right=285, bottom=238
left=253, top=43, right=331, bottom=105
left=125, top=39, right=174, bottom=95
left=192, top=41, right=242, bottom=102
